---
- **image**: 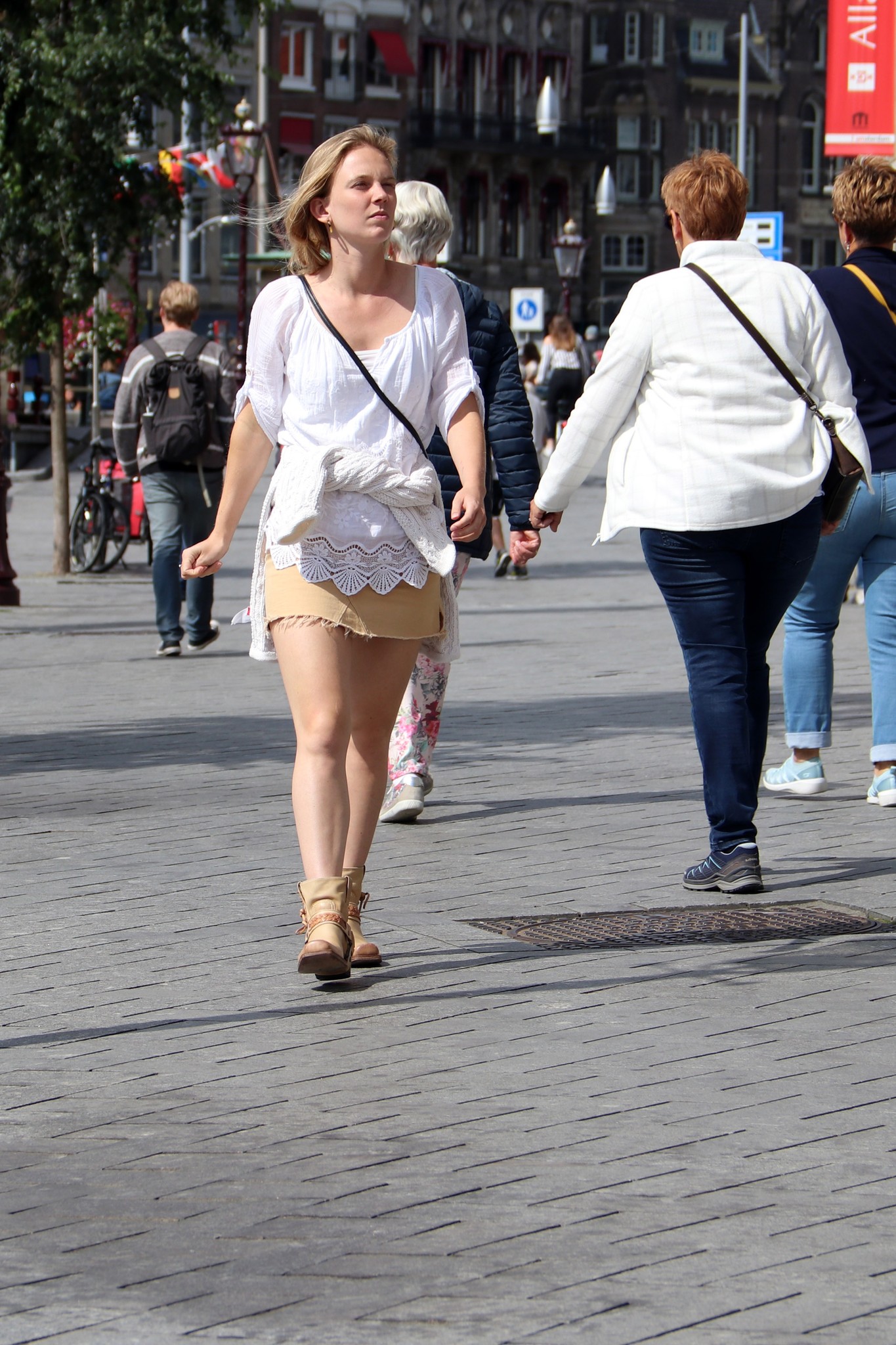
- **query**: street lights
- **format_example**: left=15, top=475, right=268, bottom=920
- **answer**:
left=550, top=217, right=588, bottom=328
left=119, top=113, right=149, bottom=361
left=220, top=96, right=267, bottom=388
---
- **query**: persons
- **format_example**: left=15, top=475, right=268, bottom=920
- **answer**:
left=179, top=122, right=490, bottom=981
left=112, top=279, right=245, bottom=658
left=529, top=146, right=873, bottom=895
left=520, top=311, right=590, bottom=455
left=759, top=155, right=896, bottom=804
left=98, top=362, right=122, bottom=411
left=376, top=180, right=543, bottom=824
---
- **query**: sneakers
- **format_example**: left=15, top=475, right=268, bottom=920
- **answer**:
left=423, top=772, right=434, bottom=795
left=762, top=751, right=827, bottom=795
left=682, top=842, right=765, bottom=894
left=188, top=620, right=221, bottom=650
left=378, top=775, right=424, bottom=822
left=494, top=550, right=511, bottom=578
left=508, top=565, right=529, bottom=579
left=156, top=639, right=181, bottom=657
left=866, top=766, right=896, bottom=806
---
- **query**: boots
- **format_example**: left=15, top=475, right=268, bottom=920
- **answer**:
left=342, top=865, right=382, bottom=964
left=297, top=876, right=355, bottom=981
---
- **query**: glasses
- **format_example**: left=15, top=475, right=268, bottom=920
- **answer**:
left=832, top=208, right=839, bottom=223
left=663, top=212, right=684, bottom=231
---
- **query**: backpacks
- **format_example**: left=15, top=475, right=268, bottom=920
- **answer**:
left=140, top=332, right=212, bottom=468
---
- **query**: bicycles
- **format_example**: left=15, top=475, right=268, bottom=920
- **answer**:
left=67, top=433, right=137, bottom=574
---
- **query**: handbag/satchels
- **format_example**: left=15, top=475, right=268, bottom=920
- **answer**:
left=821, top=427, right=864, bottom=536
